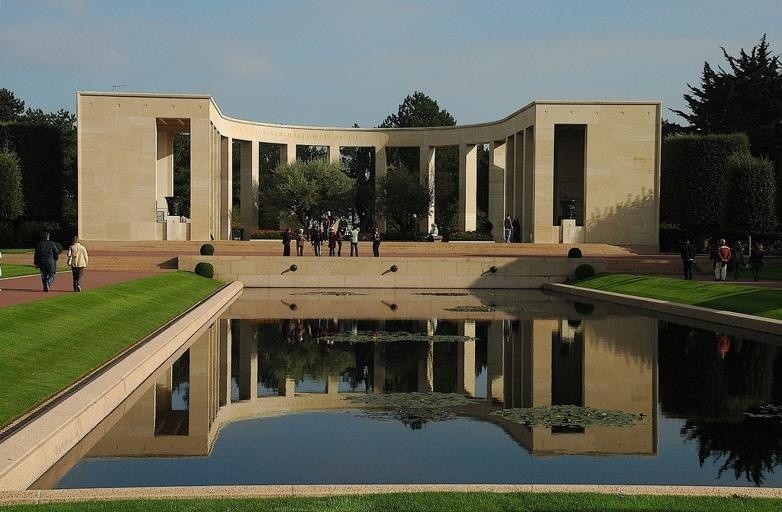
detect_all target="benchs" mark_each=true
[428,235,443,242]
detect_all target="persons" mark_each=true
[680,238,697,280]
[282,316,359,346]
[503,213,521,243]
[281,222,360,257]
[428,223,438,242]
[708,238,765,283]
[718,335,730,361]
[370,227,382,257]
[410,213,420,242]
[66,236,89,292]
[425,223,435,240]
[34,231,60,291]
